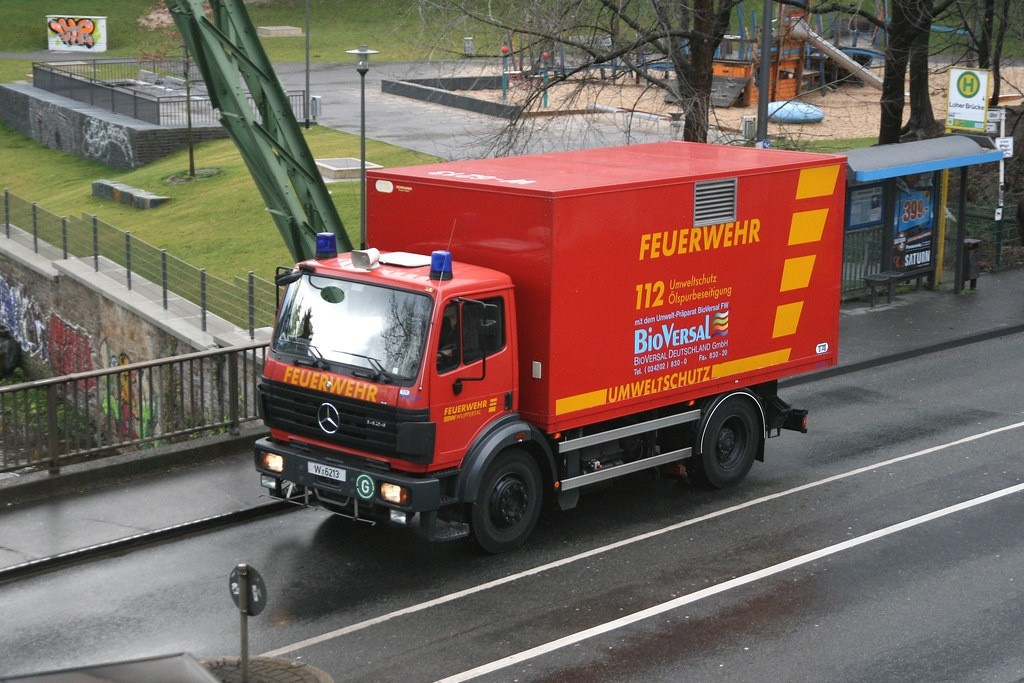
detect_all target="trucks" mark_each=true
[254,140,847,556]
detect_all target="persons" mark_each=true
[1016,198,1024,246]
[438,301,479,356]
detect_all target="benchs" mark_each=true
[860,269,905,308]
[150,76,187,96]
[125,69,159,90]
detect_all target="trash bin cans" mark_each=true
[962,239,980,290]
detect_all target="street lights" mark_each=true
[345,44,380,250]
